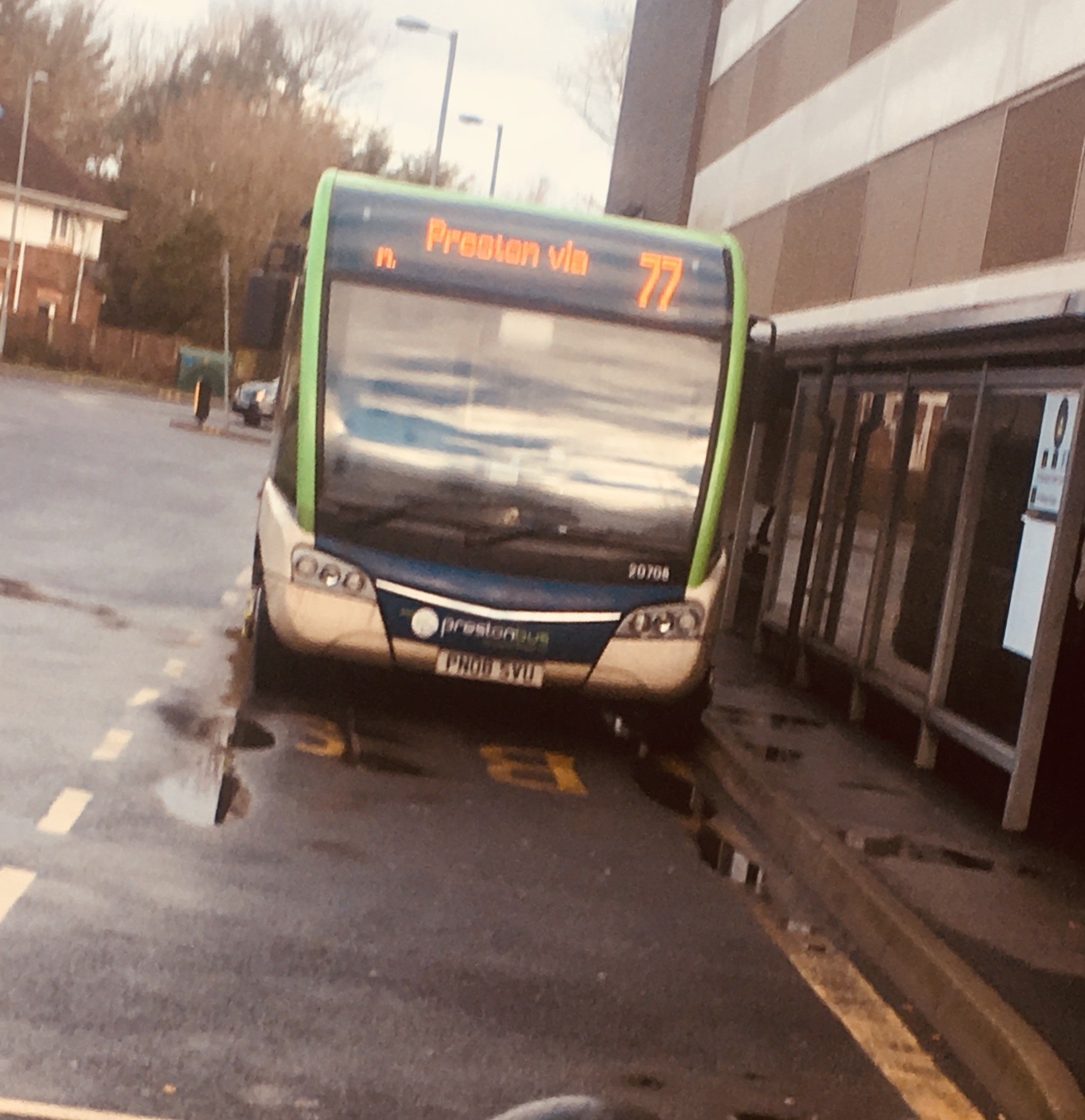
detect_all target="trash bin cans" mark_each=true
[174,346,234,398]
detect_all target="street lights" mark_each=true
[0,69,51,359]
[459,112,503,199]
[394,13,458,188]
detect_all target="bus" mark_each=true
[251,167,777,760]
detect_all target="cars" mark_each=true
[231,376,280,428]
